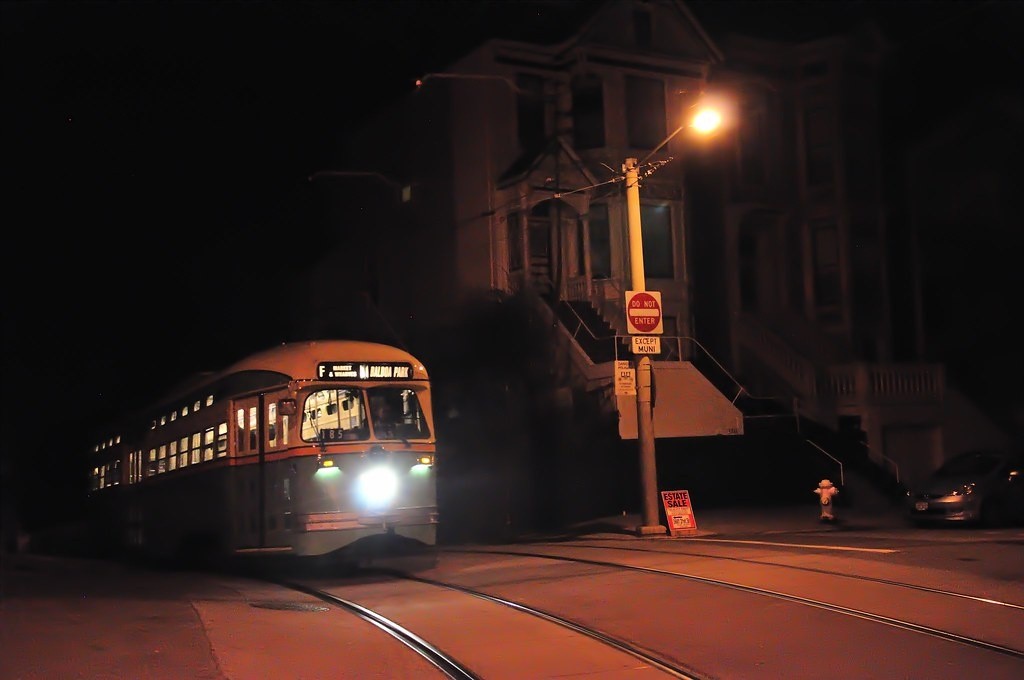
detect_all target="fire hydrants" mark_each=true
[815,479,839,522]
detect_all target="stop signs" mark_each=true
[625,291,663,334]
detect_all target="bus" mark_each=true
[83,340,444,584]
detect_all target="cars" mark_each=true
[914,458,1024,526]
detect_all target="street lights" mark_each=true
[615,92,733,533]
